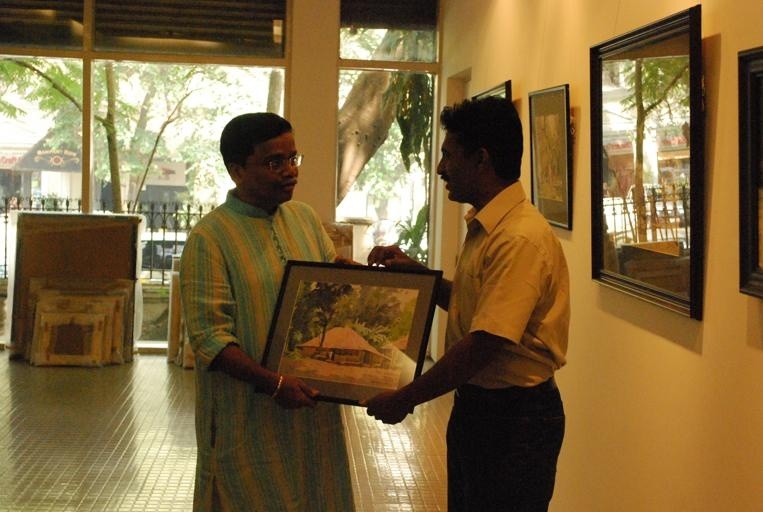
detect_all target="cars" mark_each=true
[142,232,190,280]
[603,183,691,248]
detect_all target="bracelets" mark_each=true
[270,375,283,397]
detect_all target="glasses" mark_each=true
[243,152,302,175]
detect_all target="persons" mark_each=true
[179,112,364,510]
[363,94,572,510]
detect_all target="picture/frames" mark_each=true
[527,82,576,233]
[585,2,707,323]
[8,212,141,369]
[471,76,514,101]
[166,254,196,369]
[253,260,444,414]
[734,41,763,304]
